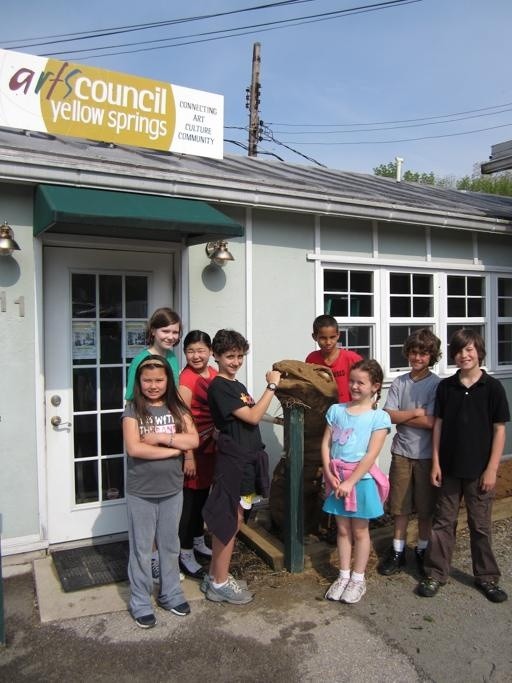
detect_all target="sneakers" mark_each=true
[418,576,441,596]
[340,578,366,603]
[135,548,255,628]
[480,581,507,601]
[327,576,349,599]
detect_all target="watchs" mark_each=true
[266,382,277,390]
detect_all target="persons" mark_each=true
[200,329,282,604]
[128,306,186,585]
[322,358,393,602]
[304,314,365,403]
[418,327,509,602]
[122,354,200,628]
[382,328,443,577]
[177,330,218,579]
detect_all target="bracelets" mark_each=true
[165,431,176,446]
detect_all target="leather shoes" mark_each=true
[382,545,405,574]
[415,546,425,575]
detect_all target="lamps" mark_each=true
[0,220,22,256]
[204,238,234,266]
